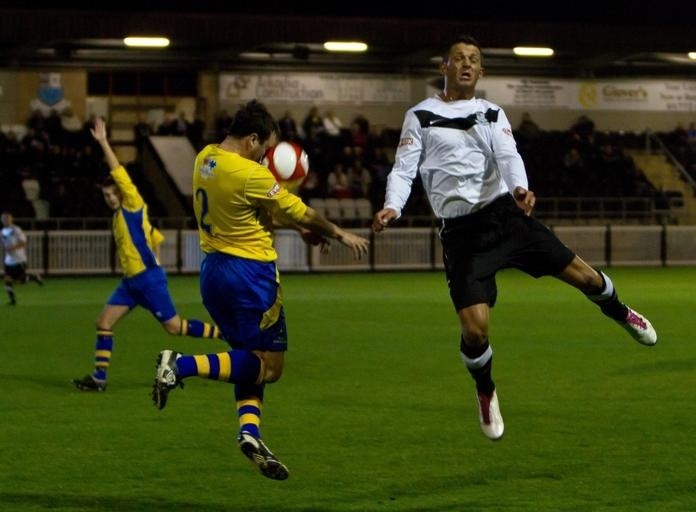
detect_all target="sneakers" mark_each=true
[239,435,289,481]
[6,299,17,305]
[35,273,43,287]
[72,376,106,393]
[610,305,657,346]
[152,350,182,409]
[474,383,504,441]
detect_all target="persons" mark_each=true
[151,99,371,479]
[278,107,431,225]
[70,117,227,390]
[371,36,658,439]
[0,213,44,305]
[0,110,232,218]
[514,111,650,217]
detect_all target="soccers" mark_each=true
[261,142,309,189]
